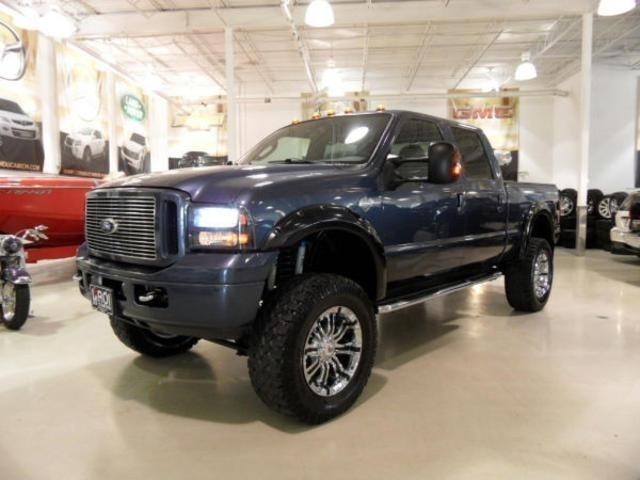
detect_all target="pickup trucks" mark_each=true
[74,109,560,424]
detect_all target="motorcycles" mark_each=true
[0,224,48,331]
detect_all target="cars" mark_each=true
[119,132,151,173]
[178,151,227,168]
[609,188,640,258]
[0,97,41,142]
[64,127,106,163]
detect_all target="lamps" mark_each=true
[514,50,538,82]
[303,0,335,29]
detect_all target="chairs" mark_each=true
[398,145,427,176]
